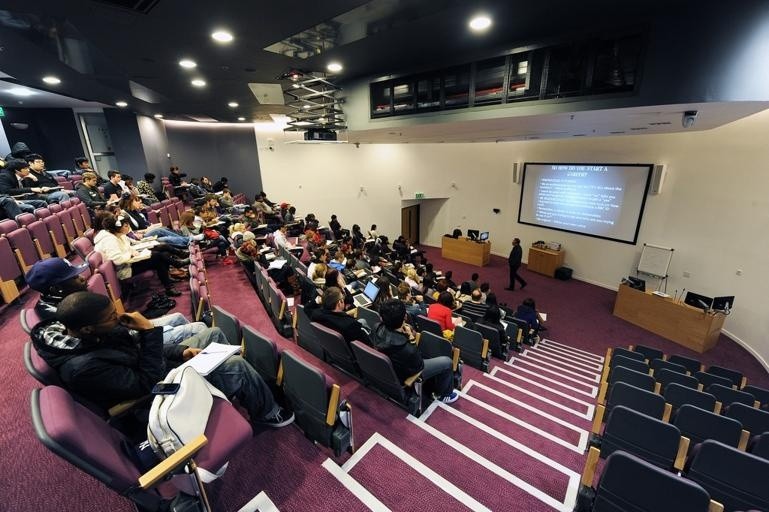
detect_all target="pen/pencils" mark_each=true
[201,350,228,354]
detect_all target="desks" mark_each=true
[527,245,566,278]
[441,234,491,268]
[612,281,726,354]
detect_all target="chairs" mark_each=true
[699,365,747,389]
[645,358,686,377]
[600,355,649,379]
[365,266,374,278]
[85,250,129,315]
[661,355,701,373]
[188,263,210,296]
[294,268,306,292]
[314,287,327,298]
[738,430,769,460]
[260,271,277,315]
[356,306,382,329]
[452,325,492,372]
[697,383,754,410]
[452,310,474,329]
[83,228,99,244]
[68,173,82,188]
[374,273,381,281]
[174,225,218,262]
[506,311,541,346]
[187,254,208,277]
[232,192,248,207]
[592,382,666,426]
[753,400,769,412]
[474,322,511,362]
[628,345,663,363]
[71,237,154,285]
[648,367,698,390]
[417,330,464,390]
[15,212,55,261]
[350,339,425,417]
[229,244,241,254]
[713,400,769,436]
[60,199,89,240]
[398,278,407,285]
[297,261,308,277]
[70,263,110,304]
[238,214,262,246]
[70,196,94,233]
[188,277,211,325]
[502,319,524,353]
[47,203,80,251]
[675,436,768,511]
[402,309,413,325]
[18,309,48,337]
[328,225,338,242]
[588,403,679,475]
[0,237,23,315]
[0,218,39,273]
[386,271,397,283]
[253,261,267,293]
[574,446,710,512]
[303,277,316,299]
[151,202,170,228]
[188,245,204,263]
[462,309,486,324]
[686,370,734,390]
[277,243,284,258]
[53,176,73,190]
[281,348,354,455]
[605,348,645,364]
[283,248,291,265]
[267,232,297,258]
[415,315,443,337]
[160,174,176,198]
[239,323,284,395]
[209,305,244,356]
[170,195,186,219]
[267,282,298,337]
[234,251,281,286]
[24,340,166,428]
[30,384,253,512]
[295,302,326,361]
[310,321,363,380]
[411,285,423,298]
[33,207,66,258]
[142,206,157,225]
[162,198,180,228]
[661,402,741,450]
[290,255,299,271]
[653,381,716,414]
[424,294,438,305]
[388,282,399,297]
[597,366,655,405]
[733,384,769,403]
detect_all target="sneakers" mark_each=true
[437,392,458,404]
[256,403,296,427]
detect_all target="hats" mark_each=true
[280,201,289,210]
[24,257,88,291]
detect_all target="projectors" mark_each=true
[303,130,338,143]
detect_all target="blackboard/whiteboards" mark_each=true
[637,242,674,279]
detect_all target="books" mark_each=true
[129,249,151,265]
[179,342,243,378]
[269,260,287,269]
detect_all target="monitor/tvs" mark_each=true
[629,276,646,292]
[684,291,713,314]
[480,232,489,242]
[712,296,735,315]
[467,229,479,241]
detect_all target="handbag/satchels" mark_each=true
[147,365,229,483]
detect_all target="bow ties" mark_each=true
[115,215,124,227]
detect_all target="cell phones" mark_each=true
[152,383,180,395]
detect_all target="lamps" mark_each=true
[0,105,7,119]
[167,153,172,159]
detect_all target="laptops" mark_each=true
[353,280,380,308]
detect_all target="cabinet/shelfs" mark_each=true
[372,53,534,115]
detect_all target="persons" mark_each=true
[74,172,107,209]
[368,298,459,404]
[5,142,29,162]
[121,174,140,197]
[99,201,193,247]
[192,176,296,261]
[32,291,294,429]
[481,282,497,306]
[25,154,70,204]
[0,159,48,213]
[468,273,479,291]
[478,304,509,345]
[523,298,547,330]
[439,271,457,292]
[456,281,471,303]
[432,280,449,301]
[169,164,188,198]
[396,287,427,329]
[466,290,486,304]
[25,257,208,349]
[427,292,455,330]
[104,169,123,199]
[138,172,160,203]
[504,238,528,292]
[70,157,109,186]
[298,214,437,287]
[304,287,362,341]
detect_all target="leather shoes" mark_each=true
[174,258,190,269]
[166,285,181,296]
